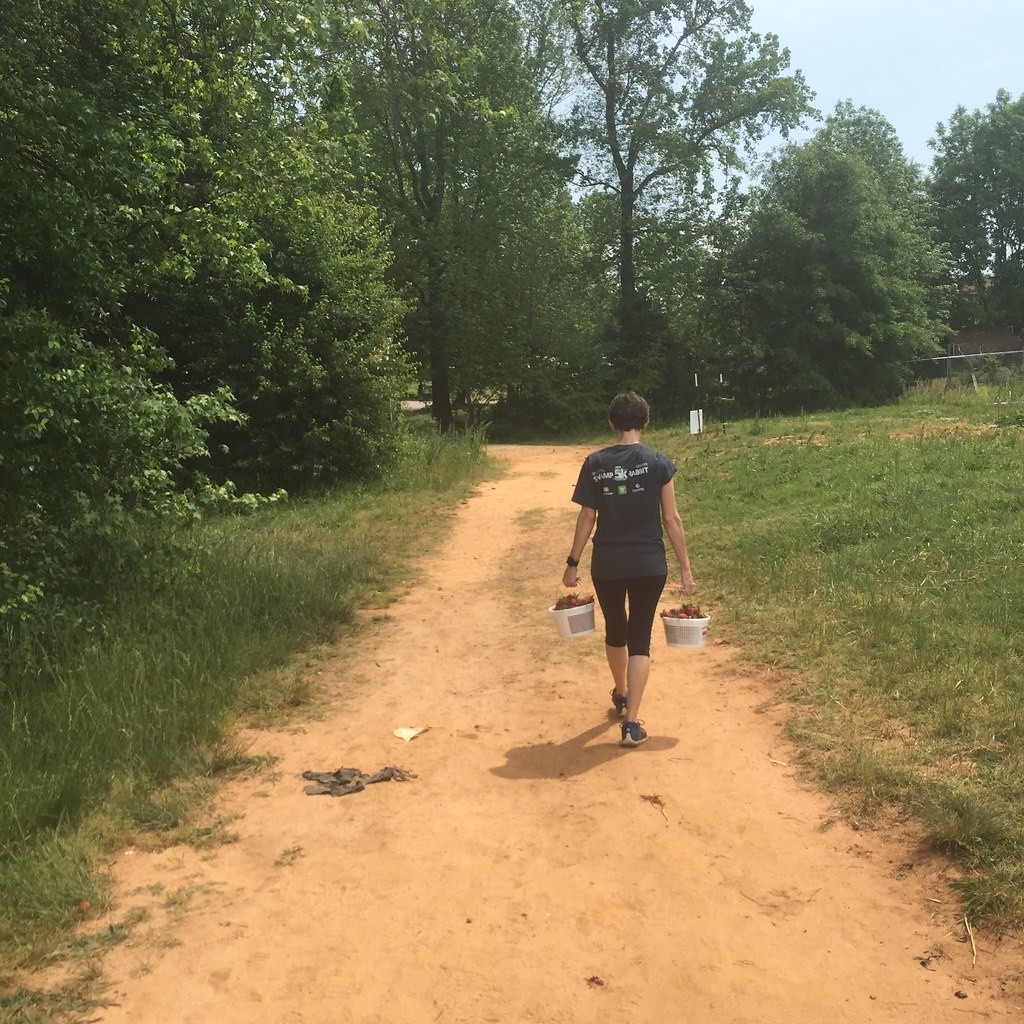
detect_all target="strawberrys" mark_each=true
[557,593,594,609]
[660,604,706,619]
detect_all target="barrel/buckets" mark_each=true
[661,590,710,648]
[548,578,596,640]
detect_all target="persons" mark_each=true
[562,391,696,747]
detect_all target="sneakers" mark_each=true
[619,719,648,745]
[609,688,627,713]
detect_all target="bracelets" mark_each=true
[565,556,580,567]
